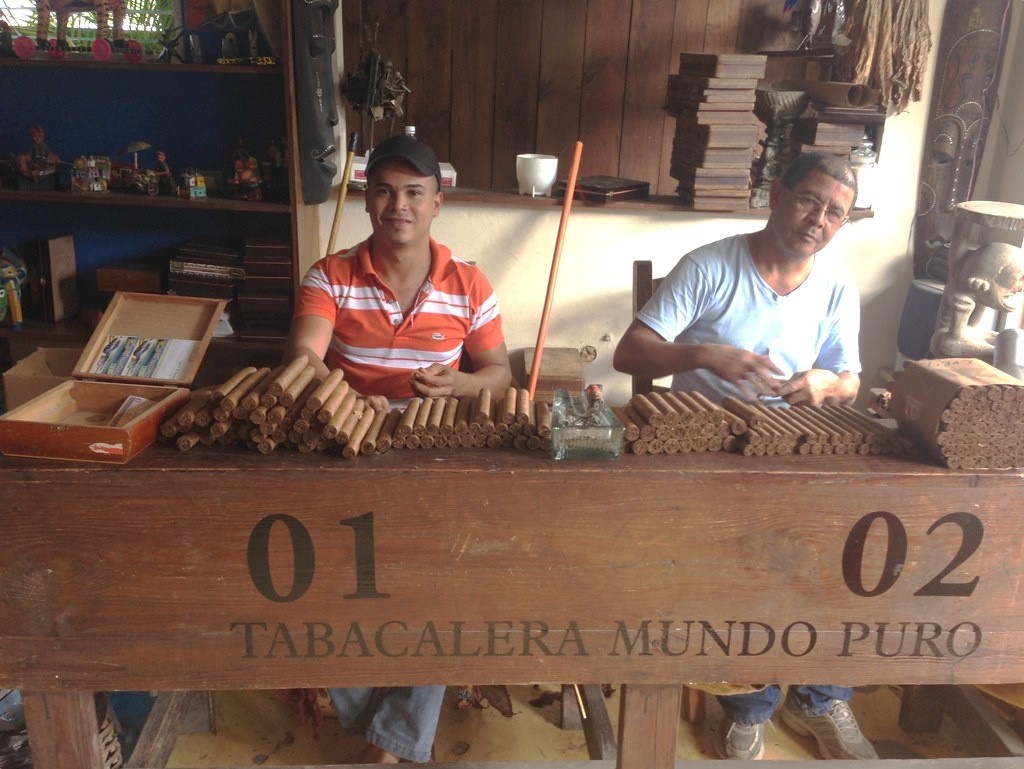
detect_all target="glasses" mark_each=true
[791,191,847,227]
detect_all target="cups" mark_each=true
[515,153,559,198]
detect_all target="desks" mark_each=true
[0,415,1023,768]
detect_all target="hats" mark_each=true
[364,135,441,192]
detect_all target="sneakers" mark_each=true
[780,691,879,760]
[714,714,766,761]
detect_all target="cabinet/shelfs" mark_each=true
[0,0,302,345]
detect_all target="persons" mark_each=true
[613,150,881,760]
[233,157,262,200]
[17,125,60,182]
[283,136,511,763]
[154,151,177,195]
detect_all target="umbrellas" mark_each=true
[118,140,151,169]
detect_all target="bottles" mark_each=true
[405,125,417,141]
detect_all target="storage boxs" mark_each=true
[0,235,290,463]
[667,53,864,212]
[524,346,586,397]
[558,176,650,206]
